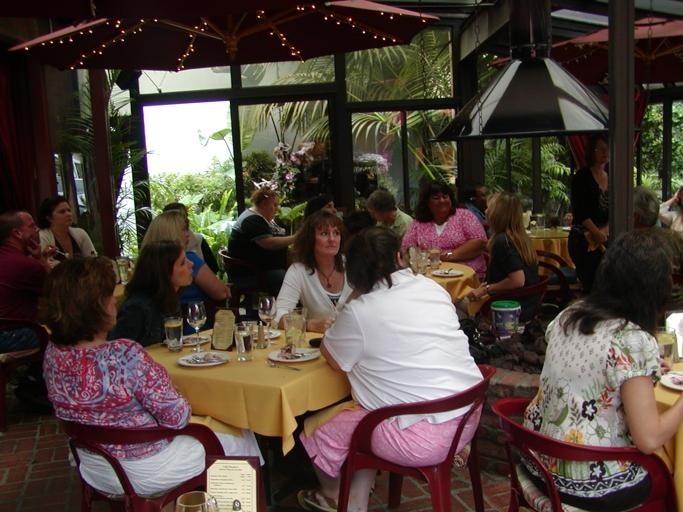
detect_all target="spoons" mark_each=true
[264,358,299,371]
[443,268,453,273]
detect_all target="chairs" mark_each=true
[1,318,52,432]
[337,364,496,512]
[491,394,681,511]
[219,250,276,322]
[56,419,228,512]
[473,249,585,356]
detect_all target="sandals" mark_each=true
[297,489,339,512]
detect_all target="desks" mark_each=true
[646,334,681,492]
[109,276,129,310]
[522,226,577,274]
[408,259,482,326]
[140,323,352,494]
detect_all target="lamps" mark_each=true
[424,0,646,149]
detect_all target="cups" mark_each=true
[409,235,440,274]
[490,300,521,336]
[283,315,305,345]
[654,330,677,366]
[233,322,254,361]
[287,307,307,346]
[529,221,538,234]
[163,316,183,351]
[114,255,131,284]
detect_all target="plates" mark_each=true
[660,372,682,391]
[252,329,280,341]
[163,336,209,346]
[431,270,463,277]
[177,352,230,366]
[561,227,571,231]
[268,348,321,363]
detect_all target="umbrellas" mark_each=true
[8,1,440,219]
[489,17,682,188]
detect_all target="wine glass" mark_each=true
[258,296,277,352]
[186,300,208,352]
